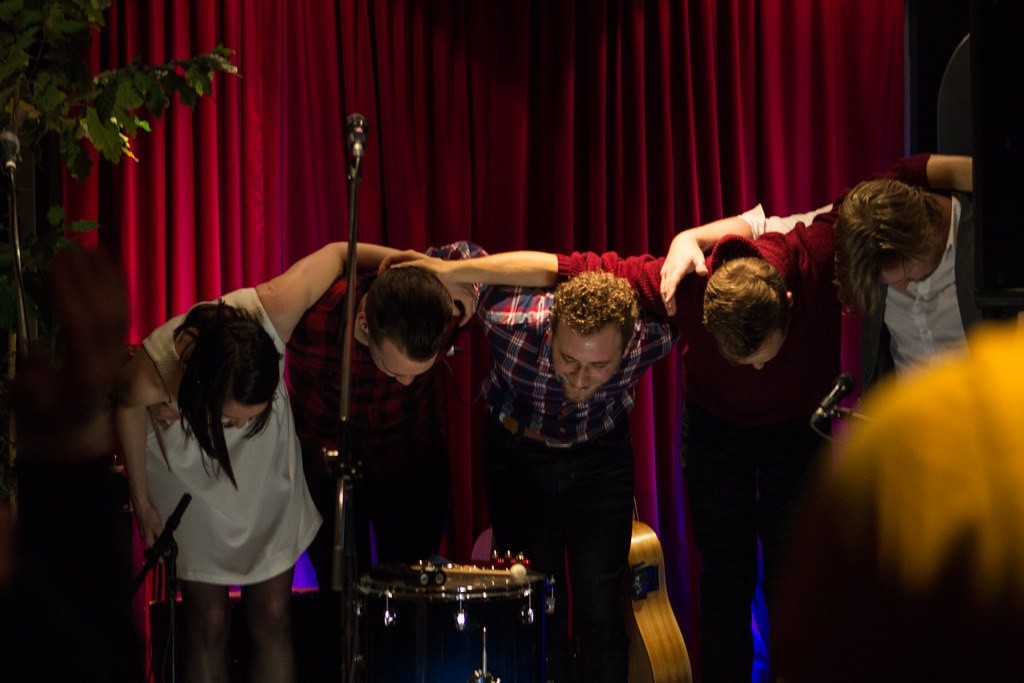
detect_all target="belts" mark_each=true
[489,406,574,446]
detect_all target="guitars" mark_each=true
[626,496,695,683]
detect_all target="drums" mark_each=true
[353,562,548,683]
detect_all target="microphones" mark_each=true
[342,113,369,181]
[0,132,21,191]
[810,374,857,425]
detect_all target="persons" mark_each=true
[376,153,973,683]
[285,240,468,683]
[659,178,1024,398]
[112,240,477,683]
[770,311,1024,683]
